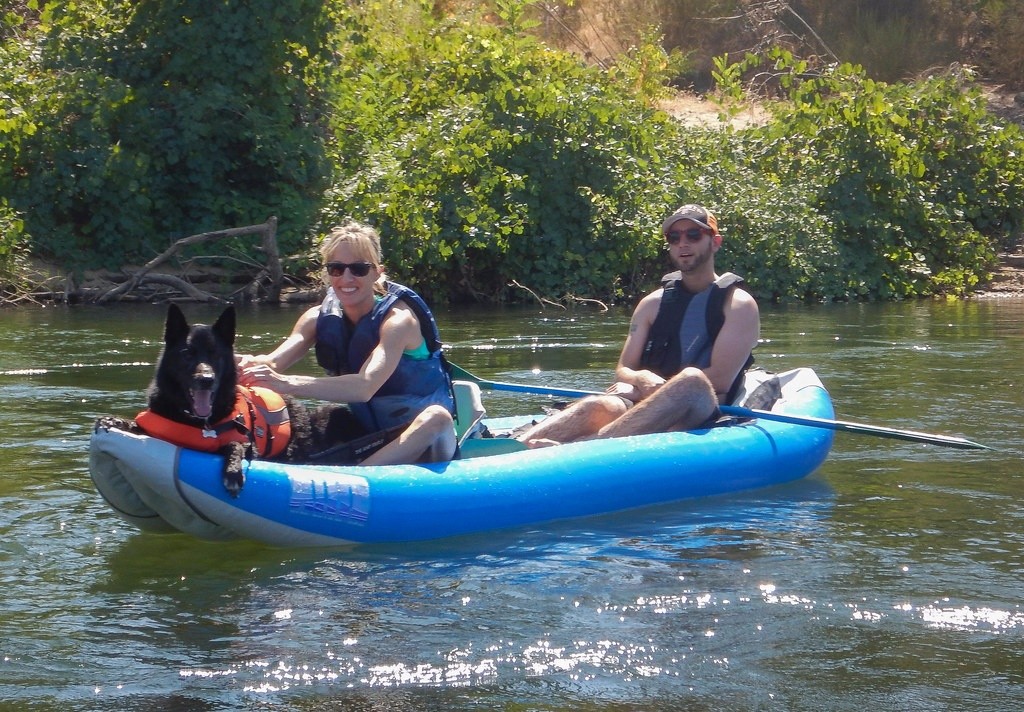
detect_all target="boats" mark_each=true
[83,361,838,555]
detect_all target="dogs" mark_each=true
[94,301,365,501]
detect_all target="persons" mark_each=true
[516,204,762,448]
[233,225,460,466]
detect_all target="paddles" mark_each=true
[446,359,992,452]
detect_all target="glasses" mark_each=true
[665,228,714,244]
[324,263,374,276]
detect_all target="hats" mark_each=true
[662,204,717,236]
